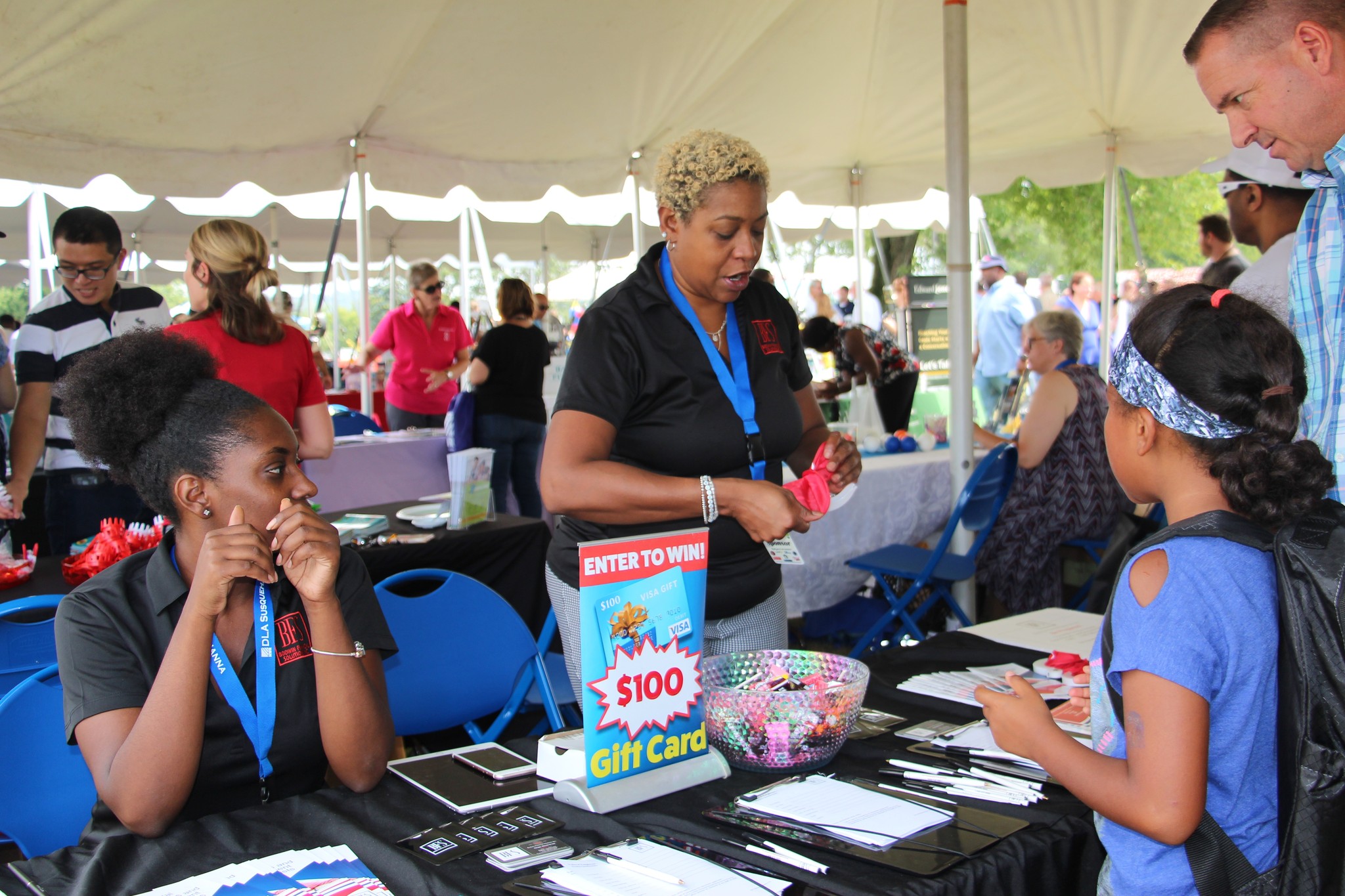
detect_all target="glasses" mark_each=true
[1026,336,1045,348]
[417,281,445,294]
[536,305,549,311]
[1216,180,1265,199]
[53,255,116,281]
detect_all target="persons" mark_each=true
[57,334,403,854]
[801,317,919,434]
[535,291,566,357]
[340,261,477,431]
[466,276,551,522]
[971,286,1310,896]
[1182,0,1345,510]
[1216,141,1317,331]
[752,266,800,327]
[974,255,1159,430]
[974,309,1138,624]
[809,279,854,320]
[539,134,867,728]
[1,206,339,561]
[1195,215,1249,289]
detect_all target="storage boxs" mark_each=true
[537,728,586,783]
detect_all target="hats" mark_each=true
[978,256,1010,274]
[1199,138,1314,190]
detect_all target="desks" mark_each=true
[0,498,552,643]
[0,607,1107,896]
[779,445,989,618]
[323,388,390,432]
[297,427,558,533]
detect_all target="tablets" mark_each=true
[385,747,557,814]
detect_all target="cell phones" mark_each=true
[451,746,537,781]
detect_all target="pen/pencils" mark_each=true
[720,837,821,875]
[741,832,833,875]
[877,745,1050,806]
[585,849,683,884]
[766,679,789,692]
[789,677,810,691]
[734,672,762,690]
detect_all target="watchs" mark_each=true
[444,369,453,381]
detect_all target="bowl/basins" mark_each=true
[702,650,870,774]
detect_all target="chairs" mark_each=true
[0,595,66,842]
[373,568,583,744]
[844,442,1018,660]
[331,411,382,437]
[1063,501,1165,611]
[0,663,97,860]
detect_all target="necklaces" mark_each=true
[657,257,727,351]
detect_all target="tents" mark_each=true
[0,174,1000,395]
[0,0,1345,426]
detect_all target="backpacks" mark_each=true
[1098,499,1345,896]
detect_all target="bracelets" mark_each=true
[700,474,719,524]
[311,641,366,658]
